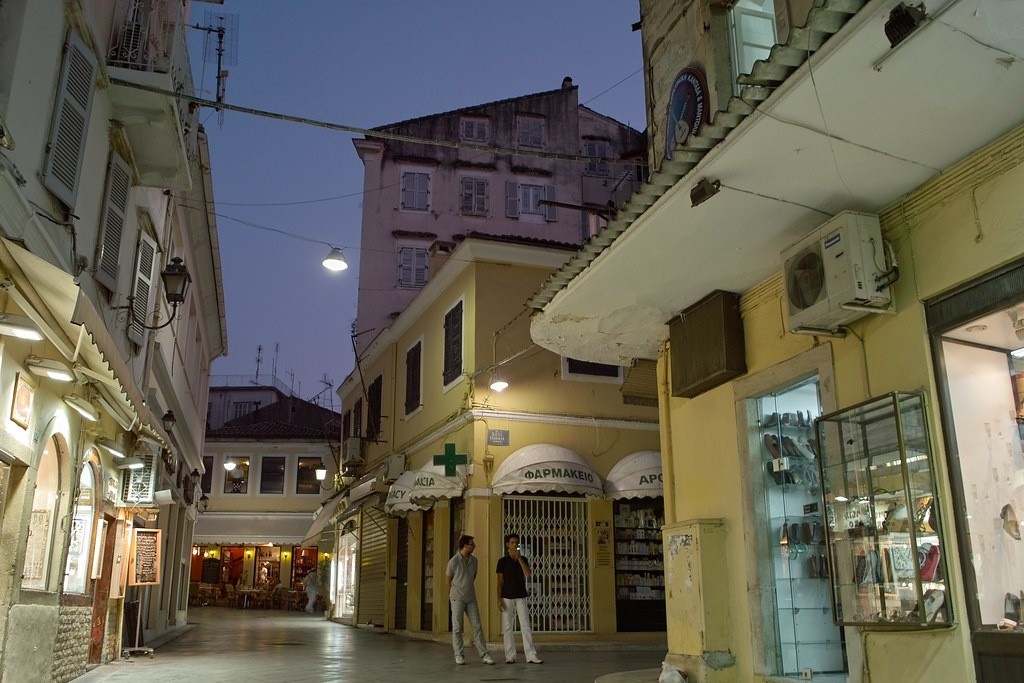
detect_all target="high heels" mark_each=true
[1000,502,1022,542]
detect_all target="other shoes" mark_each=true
[505,658,515,664]
[763,411,828,579]
[528,656,544,664]
[992,590,1024,632]
[851,498,946,626]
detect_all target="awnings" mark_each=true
[385,458,468,516]
[490,443,604,498]
[300,490,348,549]
[0,234,177,460]
[604,451,663,501]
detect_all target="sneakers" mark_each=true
[455,655,465,664]
[483,654,495,664]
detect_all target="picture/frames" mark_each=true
[11,372,38,430]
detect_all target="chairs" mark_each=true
[185,579,321,612]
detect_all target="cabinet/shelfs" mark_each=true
[814,389,954,632]
[761,422,845,647]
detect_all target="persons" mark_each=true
[446,535,496,664]
[230,465,244,493]
[303,569,319,615]
[259,563,268,582]
[496,534,544,664]
[223,565,229,581]
[260,577,284,608]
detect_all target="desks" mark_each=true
[289,590,309,609]
[236,589,261,608]
[197,587,219,605]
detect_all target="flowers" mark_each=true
[147,31,167,58]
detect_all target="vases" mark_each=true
[154,55,170,74]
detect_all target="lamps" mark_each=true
[25,356,74,381]
[689,178,722,208]
[490,371,508,391]
[181,468,202,491]
[322,246,351,270]
[195,495,211,513]
[61,395,100,422]
[224,457,236,470]
[0,311,48,341]
[315,463,338,490]
[193,544,215,559]
[114,457,144,473]
[110,257,192,331]
[94,438,127,456]
[162,409,176,435]
[247,550,252,558]
[284,552,289,558]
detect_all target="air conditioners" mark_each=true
[382,455,407,482]
[341,437,364,465]
[116,20,149,72]
[776,207,897,338]
[127,452,166,504]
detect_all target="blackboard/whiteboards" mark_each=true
[128,528,162,586]
